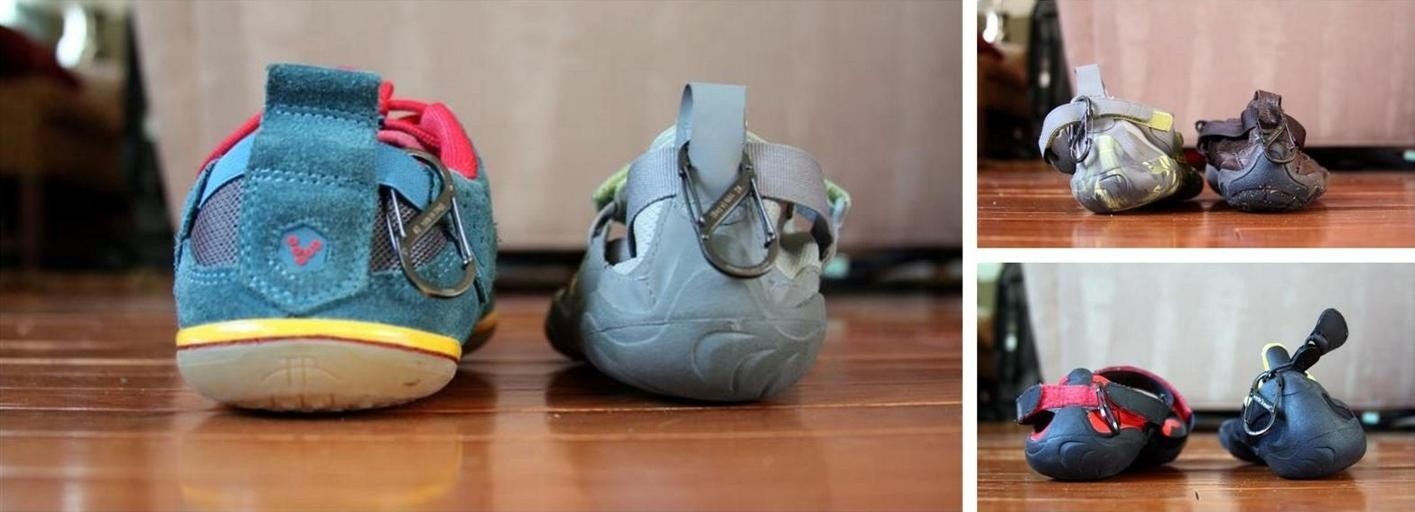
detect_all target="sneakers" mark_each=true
[1194,83,1330,213]
[1015,362,1197,481]
[542,76,854,403]
[170,63,501,417]
[1036,62,1204,215]
[1218,309,1368,480]
